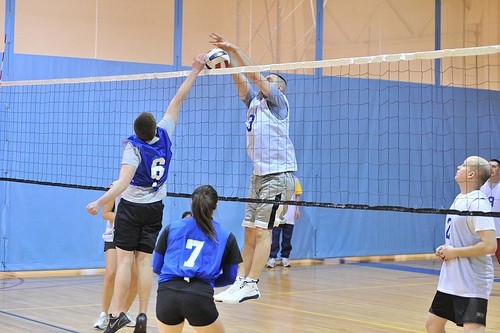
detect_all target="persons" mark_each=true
[182,211,193,219]
[209,32,298,304]
[92,180,139,330]
[423,156,497,333]
[84,52,210,333]
[479,159,500,264]
[266,174,304,268]
[150,185,244,333]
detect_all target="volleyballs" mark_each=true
[203,47,231,69]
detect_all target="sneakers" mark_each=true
[281,258,291,267]
[133,313,147,333]
[125,311,136,327]
[103,312,131,333]
[94,312,109,329]
[266,258,276,267]
[213,277,260,304]
